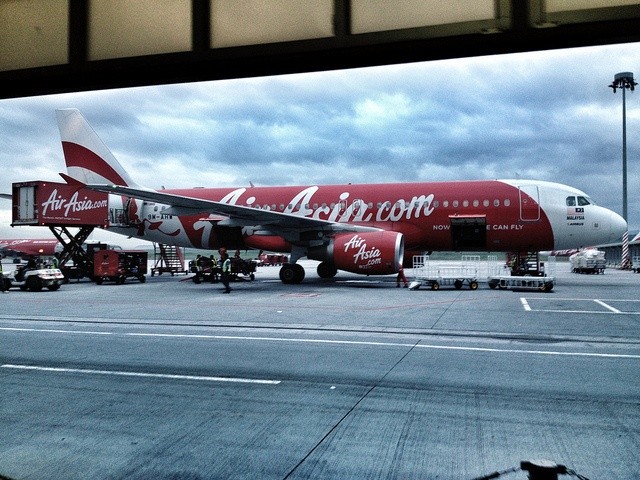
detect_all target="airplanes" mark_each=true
[10,108,628,291]
[0,239,120,259]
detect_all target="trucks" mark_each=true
[409,255,481,292]
[487,254,556,290]
[569,249,606,275]
[95,249,148,286]
[189,256,257,284]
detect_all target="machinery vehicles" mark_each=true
[150,244,188,276]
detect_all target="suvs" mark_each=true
[0,265,65,291]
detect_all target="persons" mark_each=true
[19,254,38,272]
[396,263,409,289]
[51,251,60,268]
[221,253,233,294]
